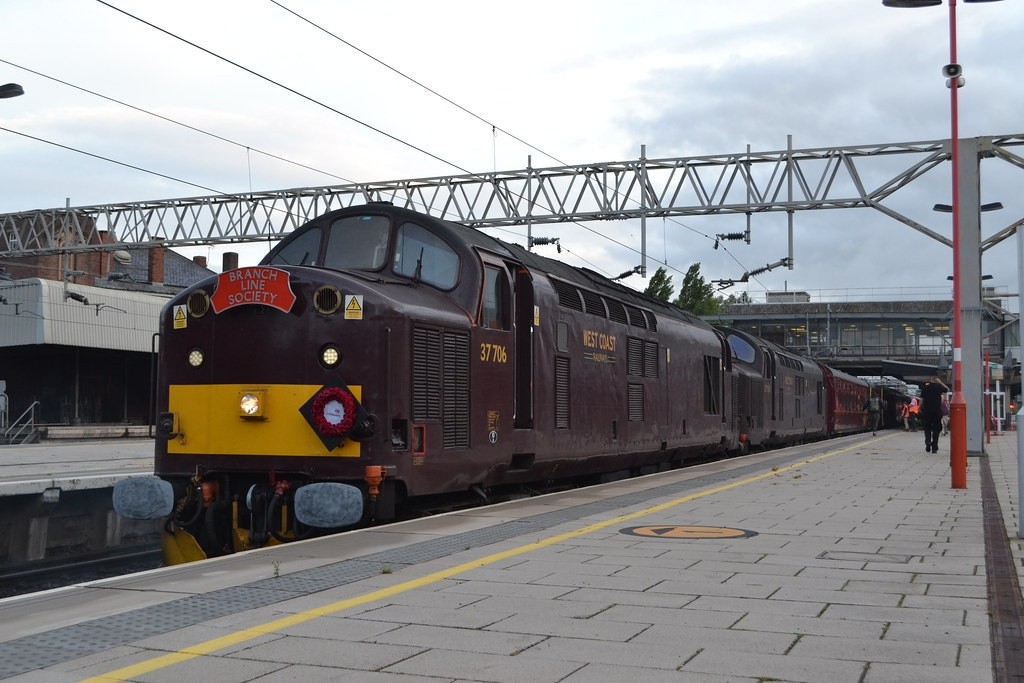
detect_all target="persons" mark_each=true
[908,397,920,432]
[920,378,949,453]
[901,401,910,431]
[863,391,883,436]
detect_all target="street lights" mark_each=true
[880,0,1005,488]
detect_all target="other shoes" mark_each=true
[926,442,930,452]
[932,450,937,453]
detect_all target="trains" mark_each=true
[110,203,921,567]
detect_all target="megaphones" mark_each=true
[945,77,965,89]
[942,64,962,77]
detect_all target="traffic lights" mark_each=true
[1010,404,1015,409]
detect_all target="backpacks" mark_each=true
[941,401,948,416]
